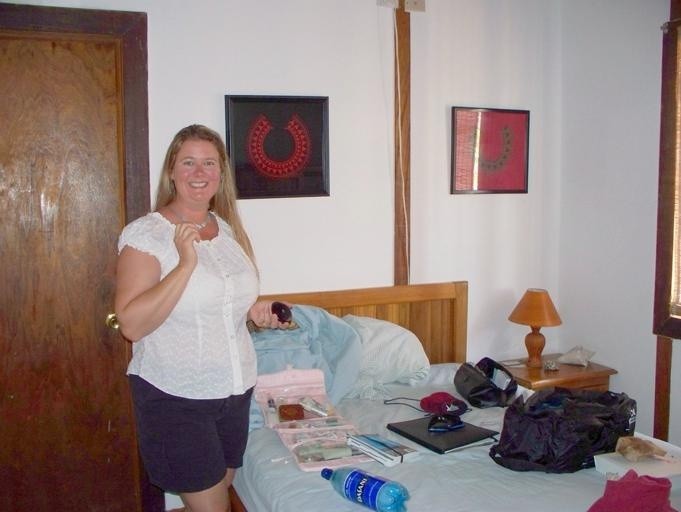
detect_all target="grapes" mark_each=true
[495,352,618,395]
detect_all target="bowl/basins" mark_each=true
[169,204,211,230]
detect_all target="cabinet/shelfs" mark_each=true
[320,466,408,512]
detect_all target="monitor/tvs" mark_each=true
[593,452,681,480]
[348,432,421,468]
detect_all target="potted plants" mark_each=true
[223,93,332,201]
[451,104,531,196]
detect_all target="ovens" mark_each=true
[228,279,681,512]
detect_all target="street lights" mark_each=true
[453,358,518,407]
[490,385,637,474]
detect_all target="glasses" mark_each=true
[508,288,563,367]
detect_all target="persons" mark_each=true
[114,124,299,512]
